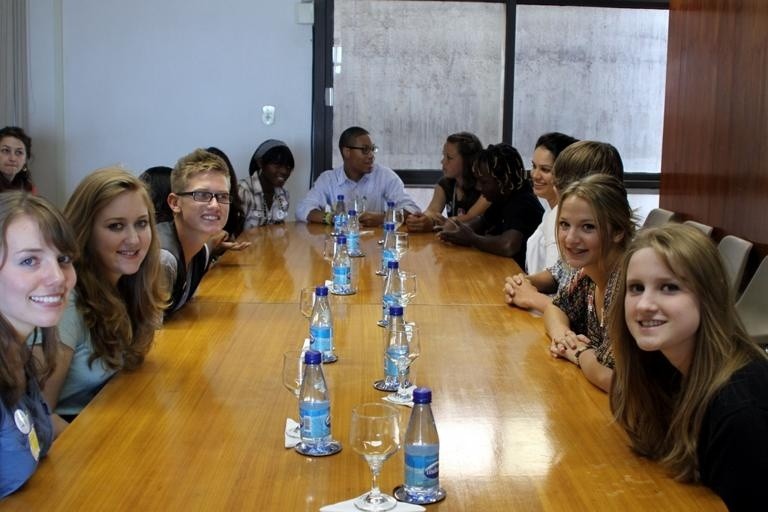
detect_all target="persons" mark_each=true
[237,139,294,230]
[522,132,580,276]
[542,172,641,393]
[405,132,494,236]
[293,126,421,227]
[430,143,543,274]
[604,221,767,511]
[206,147,253,256]
[24,166,174,442]
[154,148,232,328]
[502,138,627,314]
[0,126,37,196]
[0,187,81,501]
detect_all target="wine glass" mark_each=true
[323,231,340,285]
[300,289,327,329]
[282,349,307,438]
[383,229,409,272]
[340,195,409,268]
[391,270,417,319]
[385,321,421,403]
[349,402,401,512]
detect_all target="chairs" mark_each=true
[634,207,768,366]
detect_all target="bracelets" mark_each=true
[321,212,331,225]
[573,344,597,369]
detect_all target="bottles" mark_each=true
[298,350,331,454]
[385,306,409,388]
[330,234,351,296]
[404,387,440,500]
[382,260,402,325]
[308,287,333,361]
[329,194,398,274]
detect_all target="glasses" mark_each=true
[176,192,233,204]
[345,145,380,155]
